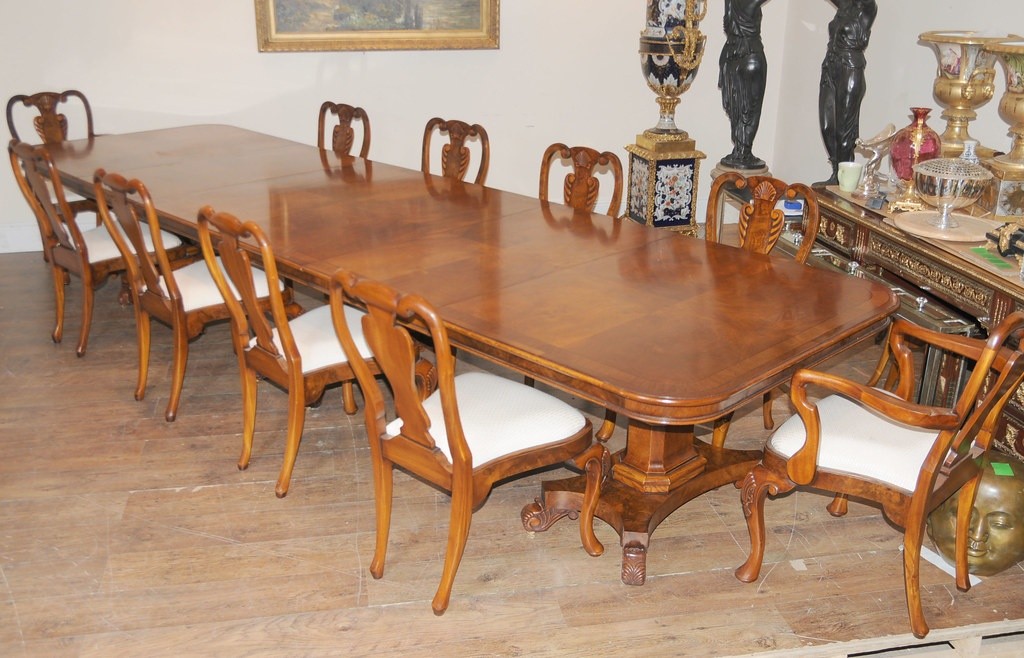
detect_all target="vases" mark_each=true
[919,29,1024,158]
[964,39,1024,222]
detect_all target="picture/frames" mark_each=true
[254,0,499,52]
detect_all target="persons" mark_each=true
[818,0,879,183]
[925,447,1024,577]
[718,0,770,164]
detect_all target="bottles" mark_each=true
[890,107,941,182]
[959,140,979,165]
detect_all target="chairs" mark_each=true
[420,116,489,185]
[8,140,187,359]
[317,100,371,157]
[7,90,113,285]
[595,171,819,447]
[197,205,437,498]
[330,269,610,616]
[92,169,306,422]
[451,142,624,389]
[734,309,1024,639]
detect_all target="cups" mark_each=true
[838,162,863,192]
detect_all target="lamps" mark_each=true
[618,0,708,236]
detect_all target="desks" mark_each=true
[791,178,1024,463]
[19,124,900,587]
